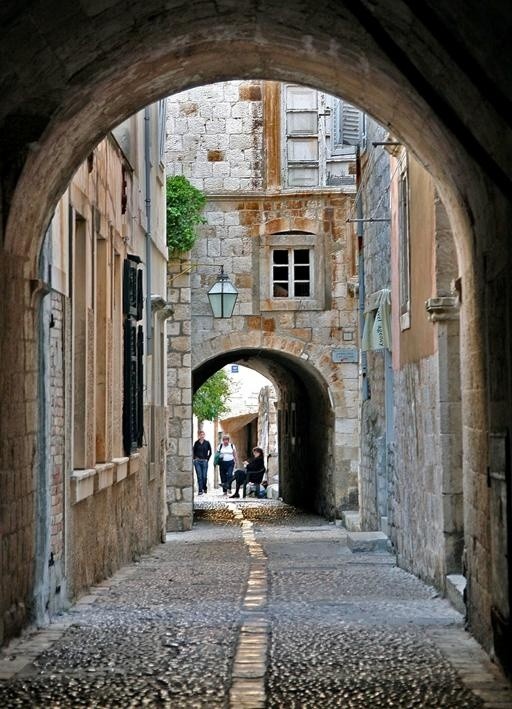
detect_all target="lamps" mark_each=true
[166,264,239,319]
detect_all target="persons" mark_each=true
[218,447,264,498]
[216,436,238,496]
[193,431,212,495]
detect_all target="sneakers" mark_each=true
[198,483,240,498]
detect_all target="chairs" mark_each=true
[243,468,264,498]
